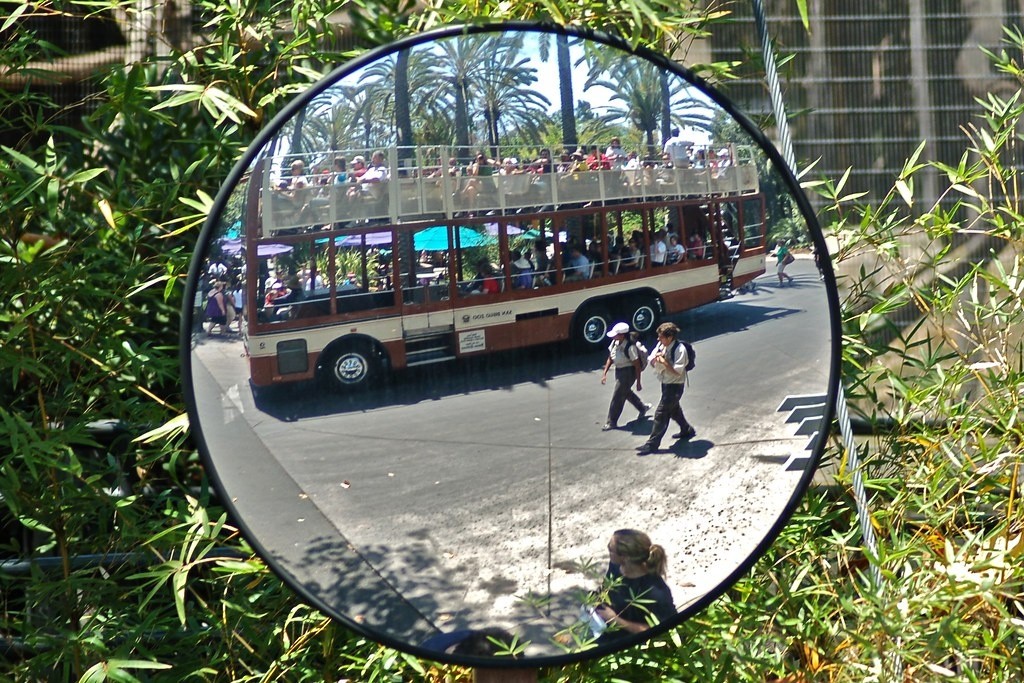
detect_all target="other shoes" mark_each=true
[636,443,658,452]
[601,423,617,430]
[776,284,783,287]
[672,426,695,438]
[787,278,793,285]
[638,403,652,418]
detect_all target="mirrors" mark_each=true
[177,19,845,669]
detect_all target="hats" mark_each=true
[606,322,630,337]
[350,155,365,164]
[716,149,727,157]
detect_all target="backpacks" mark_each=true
[624,341,648,371]
[658,341,695,370]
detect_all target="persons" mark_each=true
[601,322,649,431]
[811,243,822,280]
[771,239,793,288]
[595,529,679,644]
[635,322,695,452]
[205,127,733,338]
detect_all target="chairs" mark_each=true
[615,255,620,276]
[663,250,667,267]
[531,275,535,289]
[640,253,646,271]
[589,260,594,280]
[500,279,504,293]
[562,272,566,284]
[262,164,735,239]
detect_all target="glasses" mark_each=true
[608,543,616,554]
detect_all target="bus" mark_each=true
[241,142,766,393]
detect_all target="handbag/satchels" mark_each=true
[783,253,794,265]
